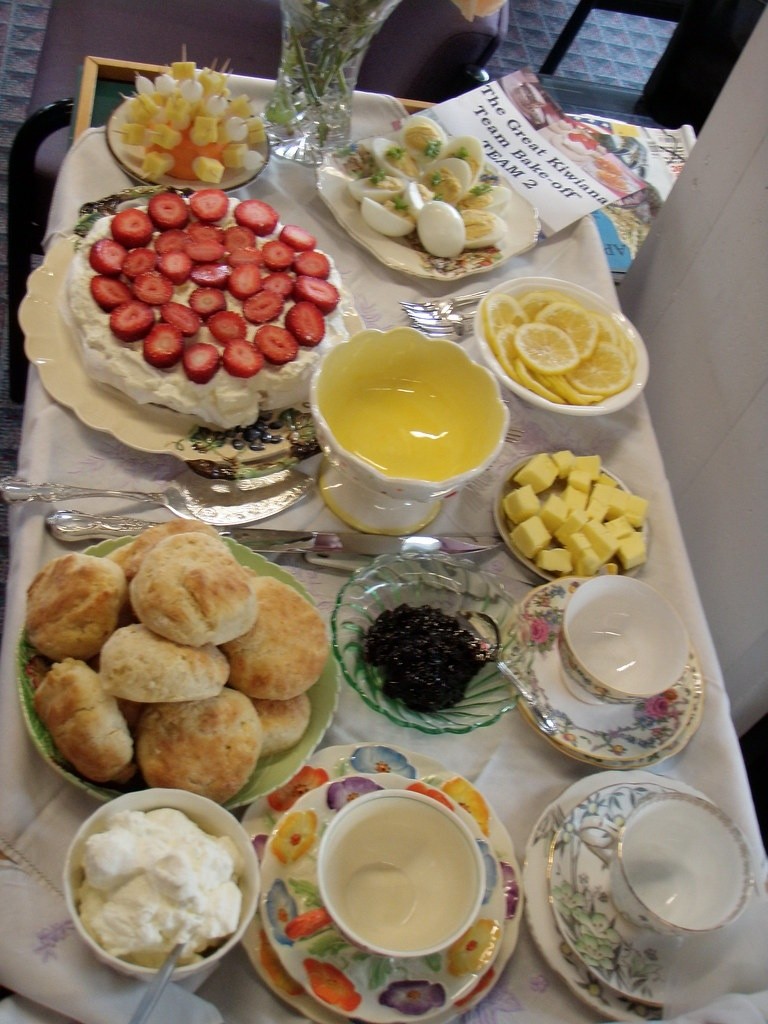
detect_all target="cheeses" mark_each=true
[504,448,648,578]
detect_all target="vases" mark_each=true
[253,0,402,165]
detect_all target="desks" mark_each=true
[2,56,768,1024]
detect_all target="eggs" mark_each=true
[346,116,510,257]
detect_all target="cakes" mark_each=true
[58,188,353,429]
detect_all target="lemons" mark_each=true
[481,289,638,406]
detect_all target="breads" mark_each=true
[23,518,330,805]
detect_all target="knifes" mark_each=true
[39,510,505,558]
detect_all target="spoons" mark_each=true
[0,470,317,525]
[455,610,561,735]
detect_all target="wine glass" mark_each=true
[309,326,512,537]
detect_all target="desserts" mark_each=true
[79,806,242,970]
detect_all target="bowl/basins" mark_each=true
[328,548,521,735]
[563,574,691,699]
[315,789,487,958]
[609,791,755,935]
[62,788,262,985]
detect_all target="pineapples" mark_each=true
[122,62,266,183]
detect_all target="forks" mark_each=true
[398,289,517,341]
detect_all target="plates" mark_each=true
[474,277,651,416]
[105,91,270,192]
[492,452,649,580]
[493,575,706,770]
[18,197,367,455]
[241,742,524,1024]
[14,535,342,811]
[522,770,768,1024]
[316,131,542,282]
